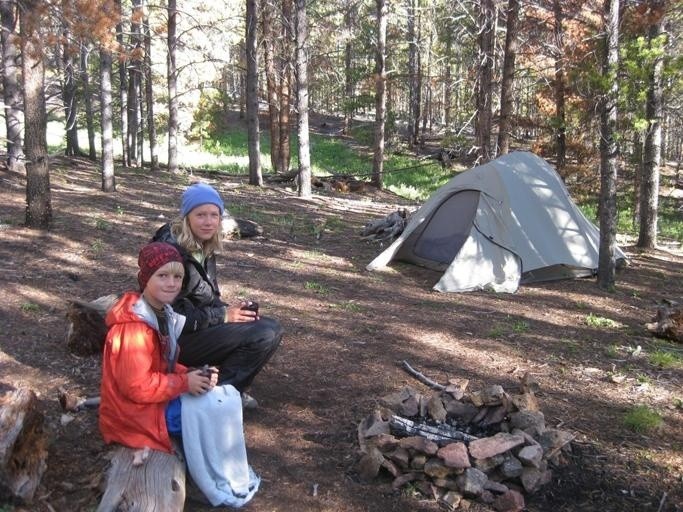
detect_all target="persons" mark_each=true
[151,183,284,411]
[97,244,243,453]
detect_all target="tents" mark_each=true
[364,150,630,295]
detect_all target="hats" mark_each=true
[182,175,224,217]
[137,241,185,294]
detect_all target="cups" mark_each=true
[199,369,212,386]
[243,301,259,319]
